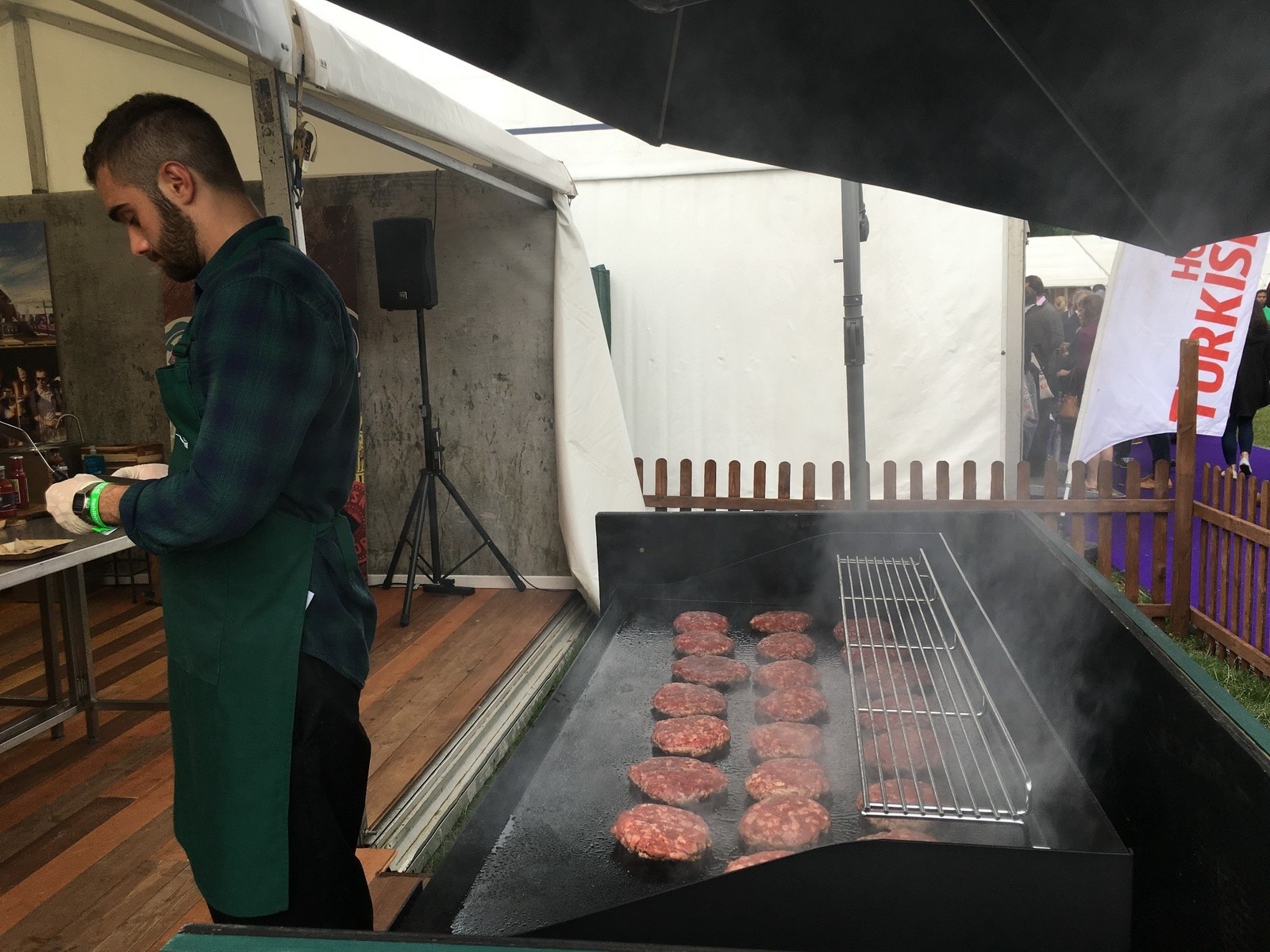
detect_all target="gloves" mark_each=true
[110,464,170,479]
[45,473,102,533]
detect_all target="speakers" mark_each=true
[373,216,439,312]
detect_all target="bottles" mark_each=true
[47,447,69,487]
[9,455,29,510]
[0,466,19,517]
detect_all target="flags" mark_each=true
[1073,232,1270,465]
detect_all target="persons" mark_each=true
[46,92,374,932]
[1022,275,1270,498]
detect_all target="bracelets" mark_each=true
[89,482,120,535]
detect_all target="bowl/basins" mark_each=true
[0,519,6,531]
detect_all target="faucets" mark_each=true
[54,414,85,443]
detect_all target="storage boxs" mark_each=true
[81,444,163,482]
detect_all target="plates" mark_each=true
[0,539,75,559]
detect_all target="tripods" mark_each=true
[380,310,527,627]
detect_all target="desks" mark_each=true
[0,515,137,754]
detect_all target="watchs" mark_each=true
[72,482,106,526]
